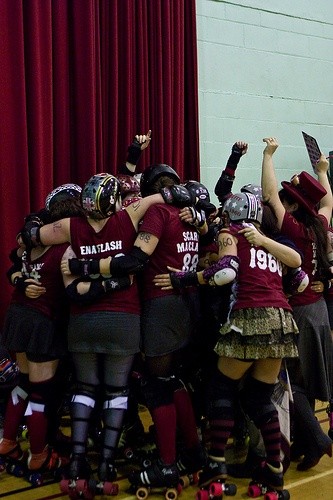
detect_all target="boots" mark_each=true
[296,416,332,470]
[288,419,320,459]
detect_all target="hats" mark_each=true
[281,171,327,216]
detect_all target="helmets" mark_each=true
[117,175,139,200]
[79,173,120,218]
[241,184,264,202]
[182,180,210,202]
[45,183,82,212]
[222,192,263,224]
[139,165,180,197]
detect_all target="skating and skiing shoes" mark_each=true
[0,424,291,500]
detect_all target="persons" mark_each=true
[0,130,333,488]
